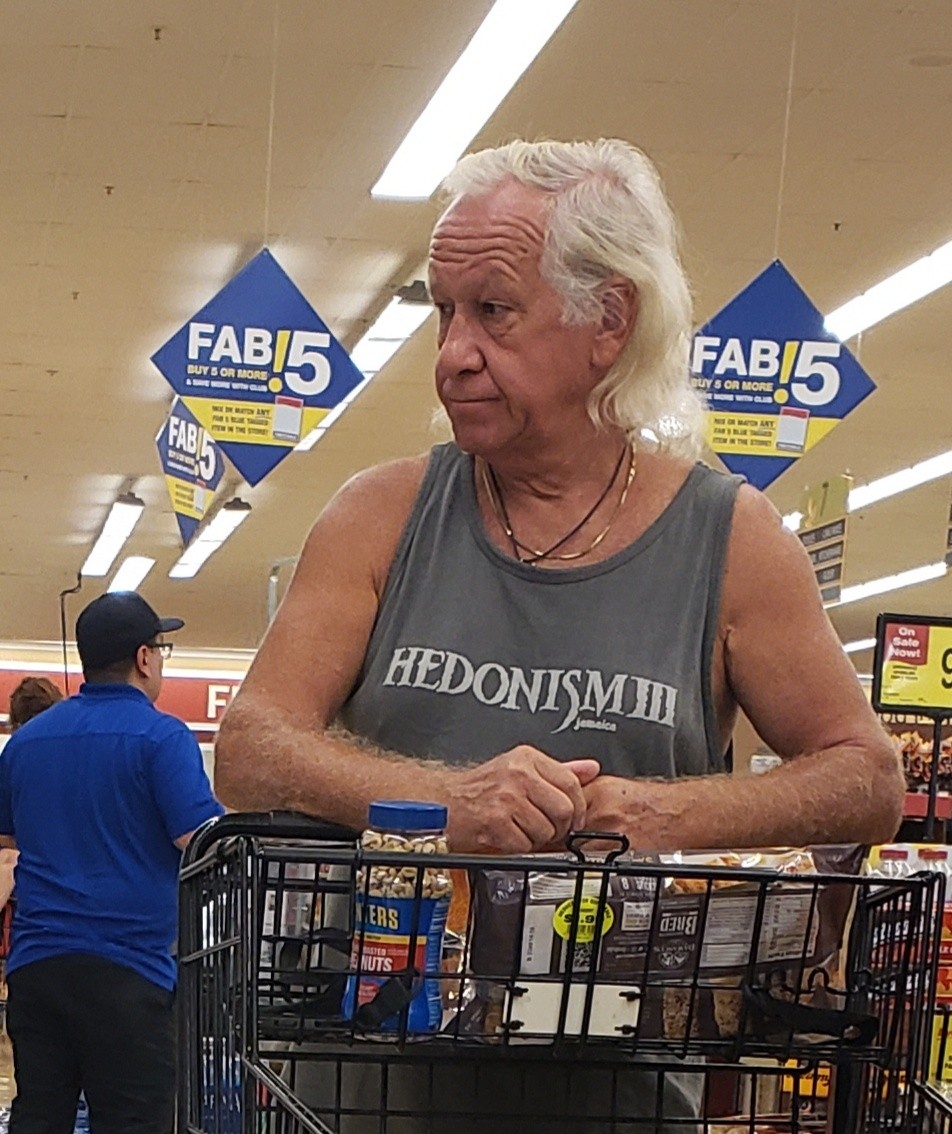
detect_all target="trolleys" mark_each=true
[175,812,952,1133]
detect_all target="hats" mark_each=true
[76,591,185,666]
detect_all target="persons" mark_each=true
[0,593,228,1134]
[214,137,907,1134]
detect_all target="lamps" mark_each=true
[294,280,437,451]
[79,491,145,576]
[167,496,251,578]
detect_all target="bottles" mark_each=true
[868,846,952,1011]
[341,801,451,1031]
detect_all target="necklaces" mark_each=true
[479,428,639,569]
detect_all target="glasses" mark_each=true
[146,642,173,659]
